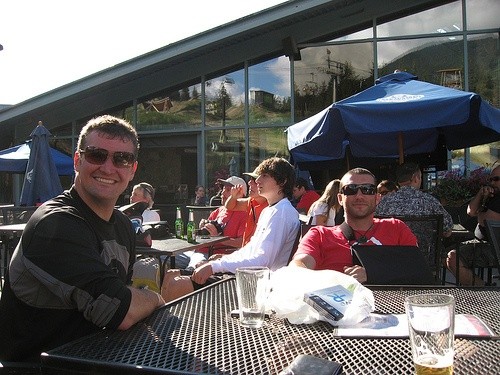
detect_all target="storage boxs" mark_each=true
[305,284,353,320]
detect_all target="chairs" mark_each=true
[373,212,444,286]
[470,219,500,285]
[0,205,38,291]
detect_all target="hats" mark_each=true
[243,166,260,178]
[218,176,247,197]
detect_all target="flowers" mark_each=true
[436,168,478,204]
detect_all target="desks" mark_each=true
[40,273,500,375]
[186,206,220,211]
[449,222,470,286]
[0,223,28,278]
[438,68,462,90]
[135,233,231,294]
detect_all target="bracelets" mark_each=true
[150,291,160,306]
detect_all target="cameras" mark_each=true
[195,220,223,235]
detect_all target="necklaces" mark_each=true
[353,223,374,243]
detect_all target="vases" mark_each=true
[441,200,463,212]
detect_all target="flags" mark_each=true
[327,49,331,54]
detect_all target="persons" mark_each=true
[190,185,206,206]
[441,165,500,286]
[228,157,239,176]
[292,176,321,214]
[196,176,255,253]
[374,163,454,261]
[160,157,300,304]
[130,183,154,246]
[306,179,341,226]
[209,167,268,260]
[0,114,169,375]
[289,168,418,283]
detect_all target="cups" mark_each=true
[404,293,455,375]
[234,266,270,328]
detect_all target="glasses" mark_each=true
[249,176,258,184]
[490,176,500,182]
[80,147,135,169]
[340,184,378,195]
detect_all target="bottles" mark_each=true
[175,207,184,239]
[187,209,196,243]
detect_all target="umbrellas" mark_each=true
[19,120,63,206]
[284,70,500,187]
[0,139,74,175]
